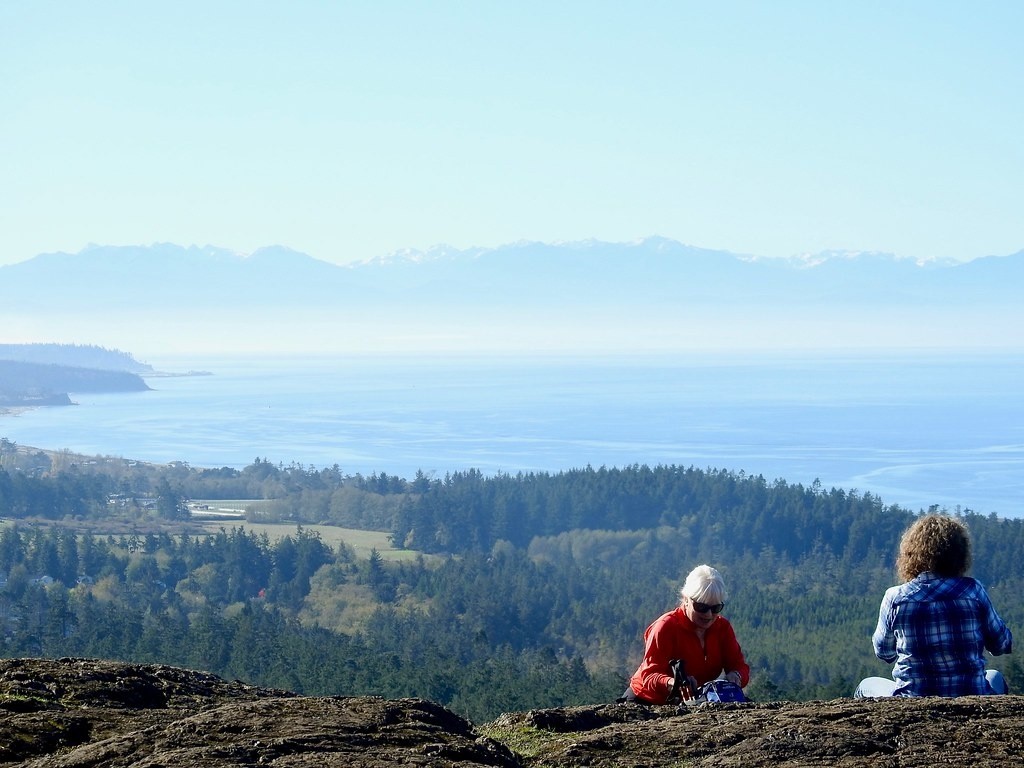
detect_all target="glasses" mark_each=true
[690,598,725,614]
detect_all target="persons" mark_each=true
[620,564,749,707]
[854,513,1012,698]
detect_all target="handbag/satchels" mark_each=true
[697,679,746,704]
